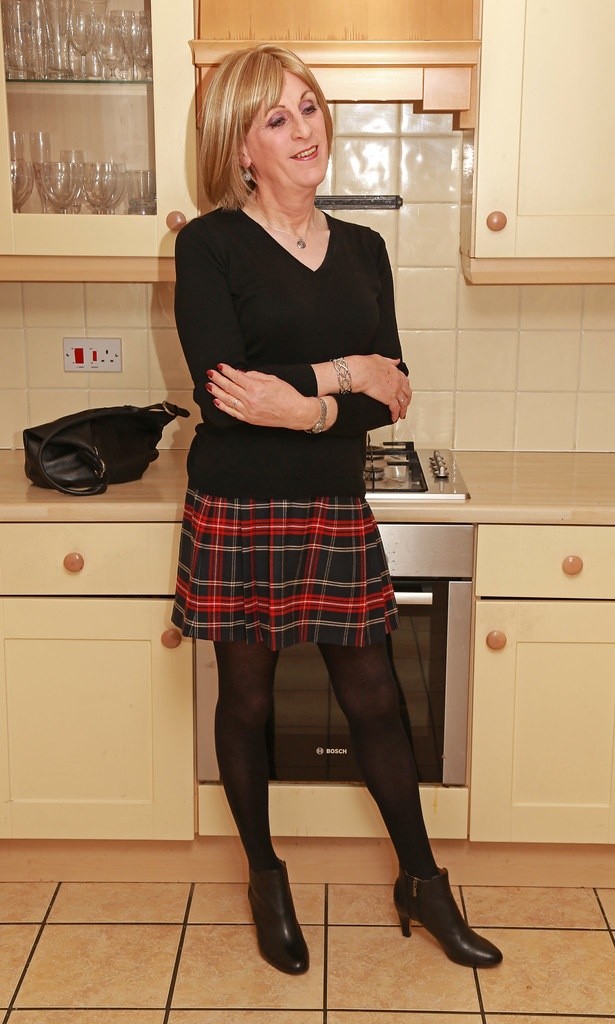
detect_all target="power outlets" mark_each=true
[63,337,122,373]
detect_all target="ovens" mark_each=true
[195,524,475,785]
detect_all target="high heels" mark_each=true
[248,857,309,975]
[393,862,503,967]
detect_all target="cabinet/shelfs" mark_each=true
[0,0,197,285]
[461,0,615,283]
[467,451,615,844]
[0,448,198,842]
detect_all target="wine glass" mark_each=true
[8,129,154,215]
[68,10,153,81]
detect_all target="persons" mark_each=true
[171,43,503,975]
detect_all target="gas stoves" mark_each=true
[363,432,471,501]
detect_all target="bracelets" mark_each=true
[306,398,327,434]
[332,357,352,395]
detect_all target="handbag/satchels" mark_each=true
[22,401,191,496]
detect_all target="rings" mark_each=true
[400,396,406,402]
[234,399,237,408]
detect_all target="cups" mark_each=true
[0,0,109,79]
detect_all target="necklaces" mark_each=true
[256,206,306,249]
